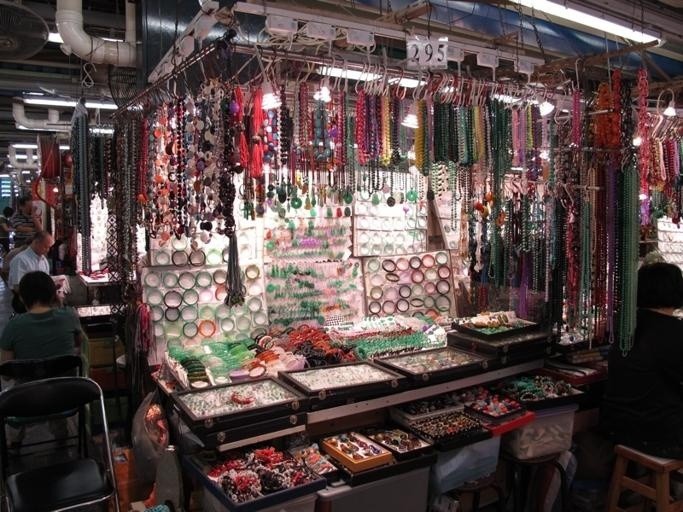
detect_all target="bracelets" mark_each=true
[180,360,267,390]
[497,374,574,402]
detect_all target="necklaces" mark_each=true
[69,68,682,359]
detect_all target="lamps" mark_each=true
[148,0,219,85]
[510,0,667,49]
[22,95,143,112]
[656,88,676,117]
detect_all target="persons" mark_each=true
[1,272,88,455]
[10,231,64,313]
[1,205,11,252]
[603,261,683,501]
[12,194,43,245]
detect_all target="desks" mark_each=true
[69,271,137,306]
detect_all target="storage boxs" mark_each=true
[197,479,317,512]
[431,434,501,494]
[577,431,611,469]
[316,464,431,512]
[508,403,578,460]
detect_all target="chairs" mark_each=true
[87,321,117,376]
[1,355,88,460]
[0,377,119,511]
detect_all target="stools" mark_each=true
[602,443,683,512]
[454,475,505,512]
[503,453,568,512]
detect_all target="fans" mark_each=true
[0,0,50,62]
